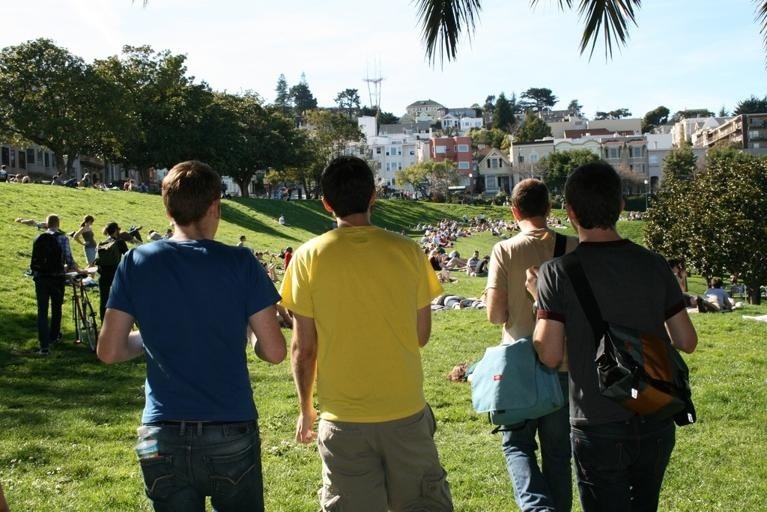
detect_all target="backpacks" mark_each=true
[98,238,119,266]
[30,233,65,277]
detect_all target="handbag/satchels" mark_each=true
[594,320,687,422]
[467,336,565,425]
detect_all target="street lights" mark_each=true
[644,178,649,208]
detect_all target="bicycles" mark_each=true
[70,272,98,352]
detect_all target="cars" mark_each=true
[389,192,404,200]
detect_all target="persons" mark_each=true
[279,215,291,227]
[96,221,173,328]
[376,183,418,200]
[669,258,744,312]
[619,212,648,221]
[221,190,233,200]
[0,164,153,193]
[417,210,520,313]
[98,161,286,511]
[524,163,699,511]
[384,225,413,236]
[488,178,580,512]
[546,213,571,230]
[276,157,452,511]
[275,303,292,328]
[28,214,292,354]
[236,235,292,281]
